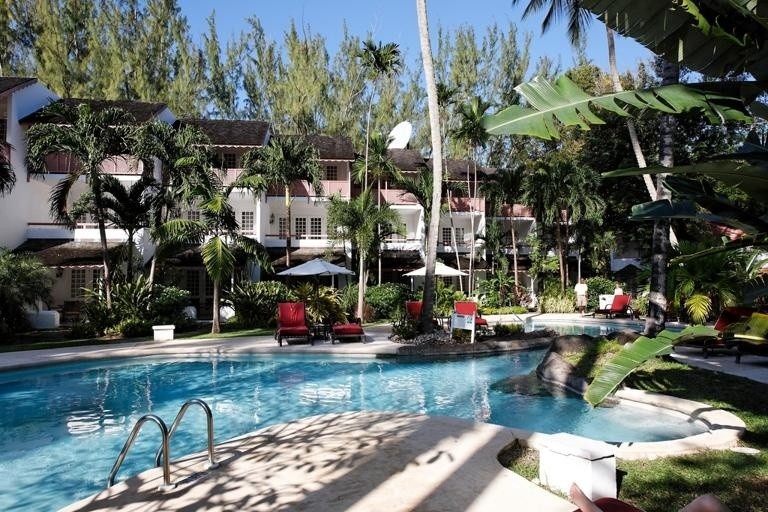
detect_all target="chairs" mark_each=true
[274,301,312,346]
[595,293,634,319]
[406,301,425,318]
[329,316,364,341]
[570,483,730,512]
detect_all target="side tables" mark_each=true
[308,322,328,337]
[433,313,450,328]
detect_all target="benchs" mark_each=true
[703,306,755,356]
[453,299,488,335]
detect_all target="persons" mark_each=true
[573,278,588,317]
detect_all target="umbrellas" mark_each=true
[278,257,355,323]
[403,262,470,310]
